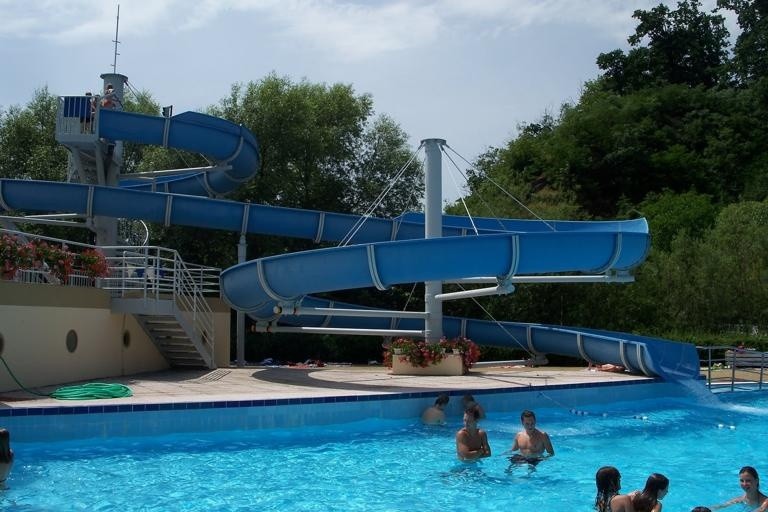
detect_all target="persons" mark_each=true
[592,466,633,512]
[421,395,450,424]
[628,473,669,512]
[80,84,120,134]
[455,408,491,460]
[506,409,554,478]
[462,394,485,419]
[710,466,768,512]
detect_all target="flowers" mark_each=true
[78,247,111,287]
[36,239,77,285]
[1,234,43,280]
[379,336,480,375]
